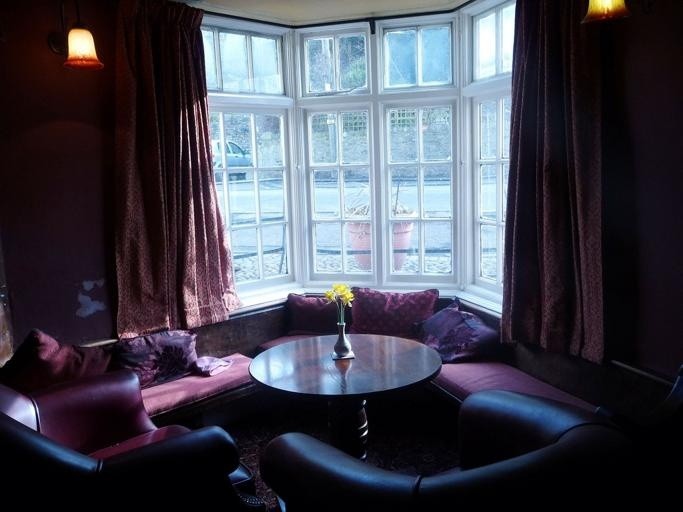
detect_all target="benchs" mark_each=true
[66,288,675,454]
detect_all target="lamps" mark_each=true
[580,0,656,25]
[45,27,106,73]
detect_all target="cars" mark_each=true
[212,141,251,182]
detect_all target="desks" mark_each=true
[246,332,442,462]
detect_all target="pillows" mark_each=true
[2,324,200,390]
[284,286,500,365]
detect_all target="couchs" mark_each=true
[3,373,254,510]
[258,388,624,511]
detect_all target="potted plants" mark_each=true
[337,179,419,274]
